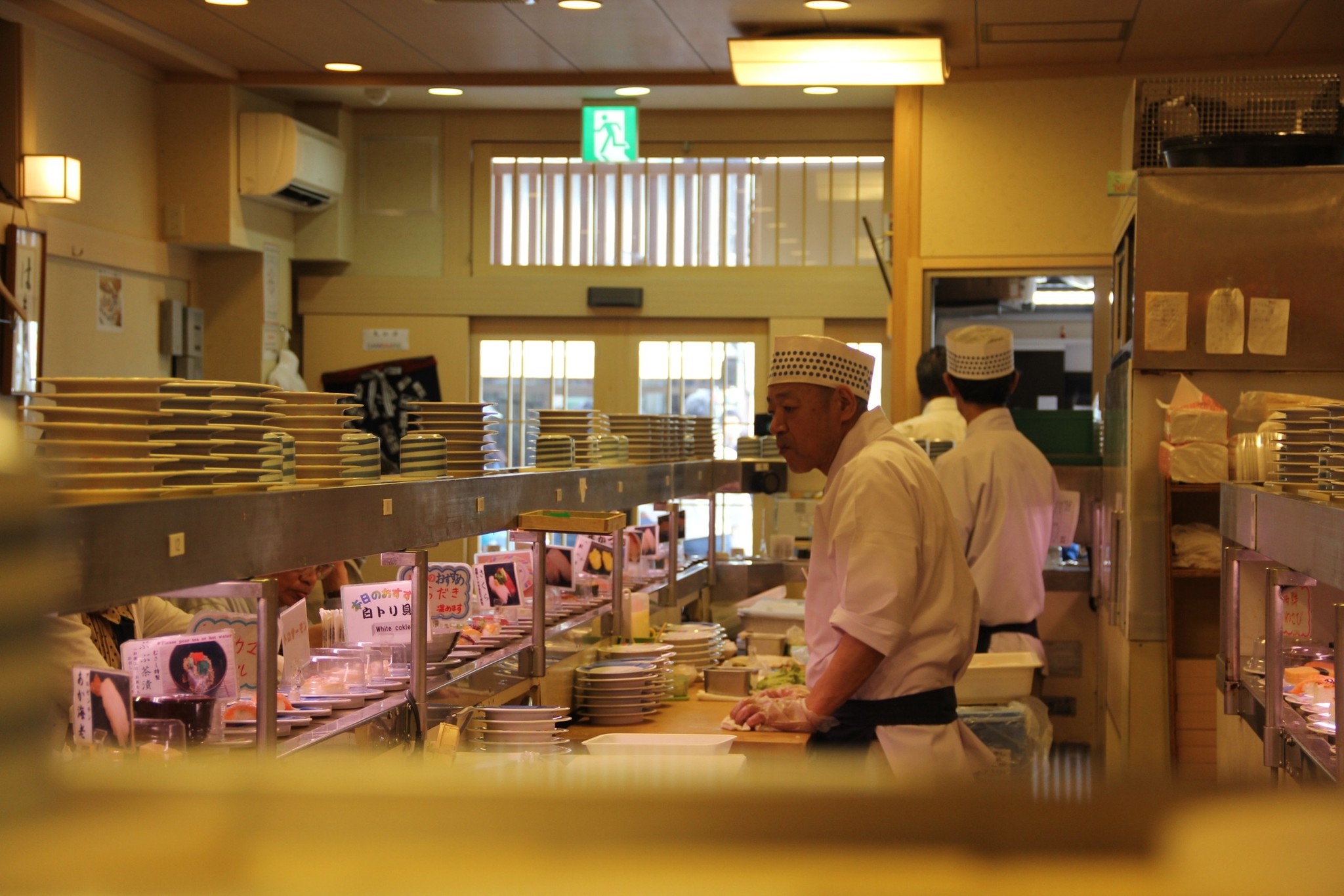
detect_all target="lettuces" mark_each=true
[754,663,806,691]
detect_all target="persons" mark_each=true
[729,334,978,755]
[177,561,350,647]
[44,595,193,751]
[893,345,967,447]
[934,325,1059,700]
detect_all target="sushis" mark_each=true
[451,616,507,646]
[587,548,613,576]
[627,528,656,562]
[224,662,387,721]
[489,568,516,605]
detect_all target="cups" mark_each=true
[535,434,573,467]
[571,438,576,467]
[258,434,282,483]
[929,440,953,466]
[279,434,296,485]
[400,433,449,478]
[617,435,630,463]
[909,439,925,451]
[593,434,620,465]
[1317,445,1331,489]
[340,433,383,480]
[737,437,785,459]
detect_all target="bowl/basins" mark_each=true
[168,642,229,694]
[131,695,222,744]
[404,629,465,661]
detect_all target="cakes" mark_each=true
[1283,662,1335,701]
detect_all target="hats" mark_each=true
[945,325,1015,380]
[766,334,876,404]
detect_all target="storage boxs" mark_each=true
[1175,657,1218,786]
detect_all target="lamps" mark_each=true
[722,29,958,94]
[17,152,85,209]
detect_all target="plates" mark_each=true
[447,558,698,658]
[425,701,465,726]
[1264,403,1344,501]
[15,376,717,504]
[225,658,460,738]
[581,733,737,757]
[1243,648,1336,738]
[573,619,727,723]
[476,706,574,757]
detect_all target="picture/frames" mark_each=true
[0,223,50,399]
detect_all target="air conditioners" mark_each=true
[235,107,350,215]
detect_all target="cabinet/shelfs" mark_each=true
[1159,476,1222,785]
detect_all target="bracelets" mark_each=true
[323,590,341,599]
[801,698,829,721]
